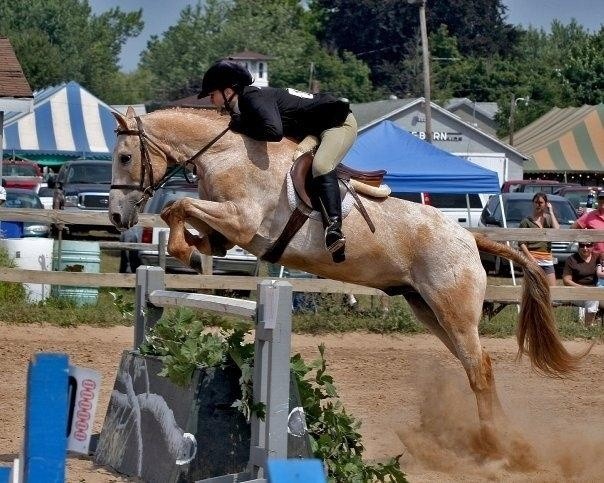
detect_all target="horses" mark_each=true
[107,106,597,456]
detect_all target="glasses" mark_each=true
[579,243,593,249]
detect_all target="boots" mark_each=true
[312,169,346,263]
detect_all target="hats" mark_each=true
[597,190,604,198]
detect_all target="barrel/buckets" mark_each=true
[1,238,30,301]
[24,236,55,305]
[52,239,107,311]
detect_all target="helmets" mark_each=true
[196,60,253,99]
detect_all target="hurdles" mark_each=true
[94,265,324,482]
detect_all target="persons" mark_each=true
[194,59,359,264]
[569,191,603,266]
[516,190,561,286]
[561,241,603,325]
